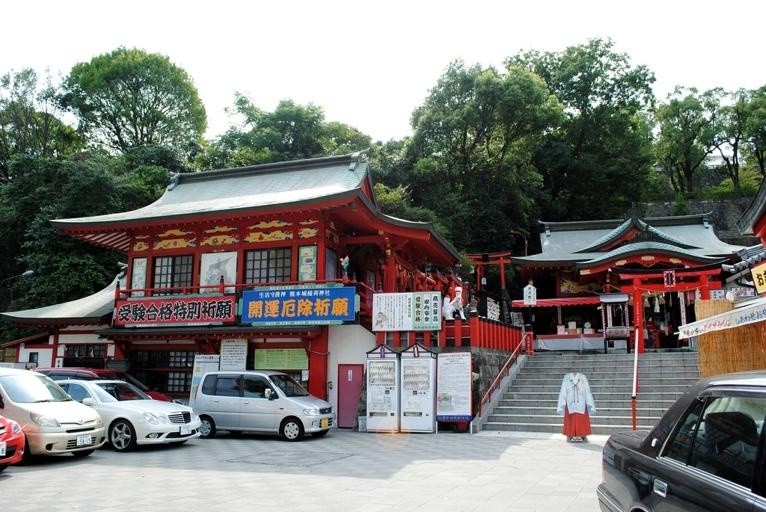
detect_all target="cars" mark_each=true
[596,369,766,512]
[0,362,204,472]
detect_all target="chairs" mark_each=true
[695,411,759,489]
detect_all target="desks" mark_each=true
[536,333,606,355]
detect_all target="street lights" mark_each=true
[510,229,529,257]
[0,270,34,283]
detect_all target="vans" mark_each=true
[194,369,335,442]
